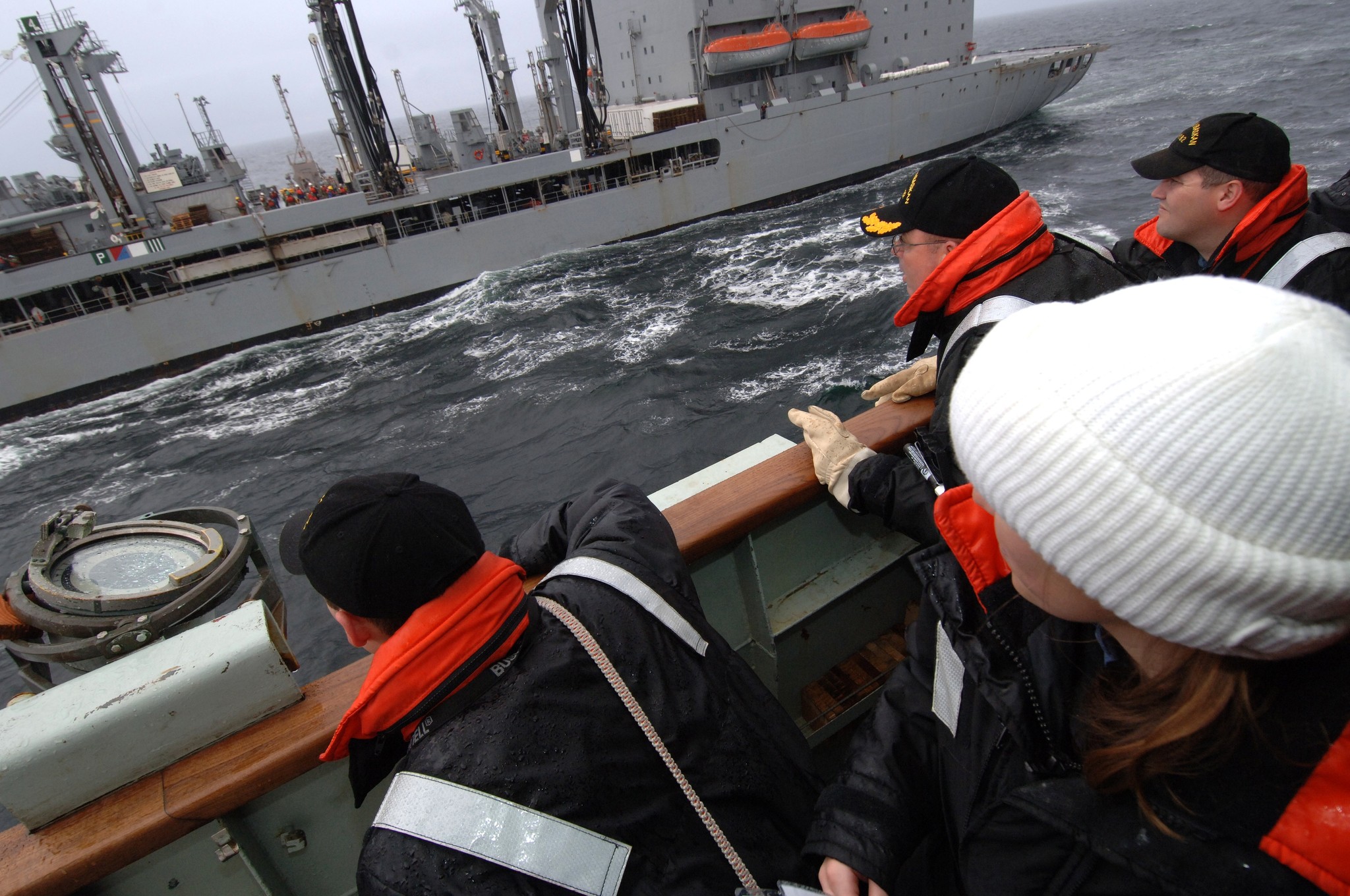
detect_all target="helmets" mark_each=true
[260,168,342,202]
[235,197,240,200]
[763,102,765,104]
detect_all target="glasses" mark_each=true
[891,232,947,255]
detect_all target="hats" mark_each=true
[860,155,1020,240]
[947,275,1350,662]
[1130,112,1290,182]
[280,475,485,618]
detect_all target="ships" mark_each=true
[0,0,1101,427]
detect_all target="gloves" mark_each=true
[861,355,937,407]
[788,405,878,516]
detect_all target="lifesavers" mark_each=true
[474,151,483,161]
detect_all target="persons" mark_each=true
[785,156,1135,545]
[103,116,710,247]
[282,470,846,896]
[812,270,1350,896]
[1113,111,1350,314]
